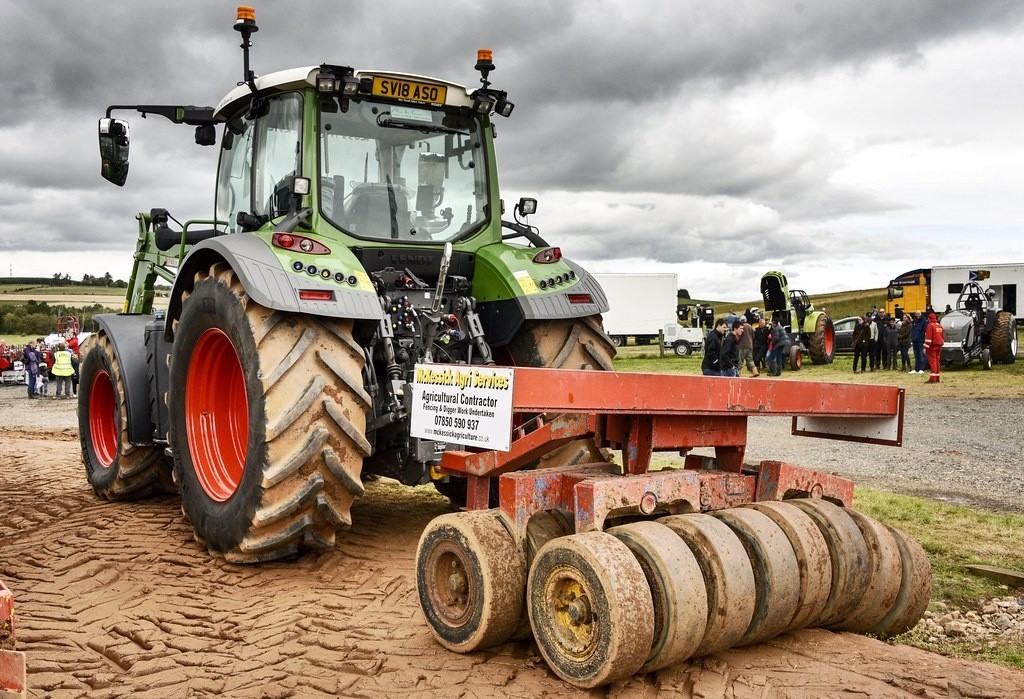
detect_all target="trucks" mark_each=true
[591,272,678,347]
[883,262,1024,320]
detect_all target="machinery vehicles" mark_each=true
[940,281,1019,370]
[750,271,835,372]
[77,4,621,567]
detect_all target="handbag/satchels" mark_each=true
[21,357,30,365]
[851,340,857,348]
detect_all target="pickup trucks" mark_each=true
[831,315,861,353]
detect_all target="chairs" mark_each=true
[348,183,411,232]
[319,175,346,220]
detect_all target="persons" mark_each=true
[945,305,952,314]
[852,310,927,374]
[895,304,904,321]
[66,332,81,356]
[692,304,700,327]
[872,305,878,316]
[724,309,738,328]
[701,319,728,377]
[719,321,745,377]
[737,316,791,377]
[926,305,934,314]
[923,313,944,383]
[24,337,79,400]
[879,309,885,317]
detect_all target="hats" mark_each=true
[772,317,778,324]
[739,316,747,322]
[928,313,937,322]
[866,312,872,317]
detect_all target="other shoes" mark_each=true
[910,365,912,371]
[885,367,890,370]
[861,369,866,373]
[876,365,881,369]
[28,391,77,400]
[894,367,899,370]
[871,367,874,371]
[853,370,857,373]
[768,371,780,376]
[899,367,906,371]
[917,370,924,374]
[908,370,917,374]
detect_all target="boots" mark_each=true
[936,374,939,382]
[929,374,936,383]
[752,367,759,377]
